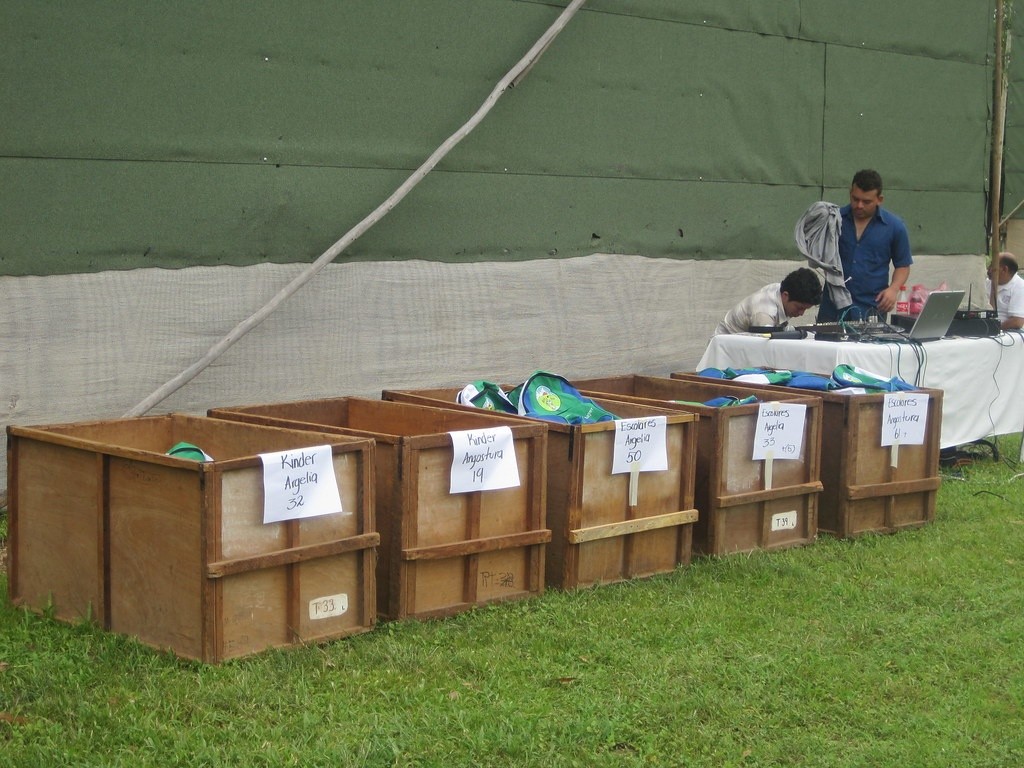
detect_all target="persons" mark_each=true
[710,267,822,334]
[986,250,1024,331]
[795,169,913,322]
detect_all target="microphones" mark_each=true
[770,329,808,340]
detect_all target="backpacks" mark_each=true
[161,442,213,465]
[672,364,915,409]
[455,371,623,430]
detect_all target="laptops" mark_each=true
[871,291,966,342]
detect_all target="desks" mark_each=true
[695,331,1024,462]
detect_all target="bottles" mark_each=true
[910,285,922,314]
[896,285,908,315]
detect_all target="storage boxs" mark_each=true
[208,394,548,621]
[383,383,700,592]
[6,414,381,665]
[671,366,942,543]
[571,364,824,559]
